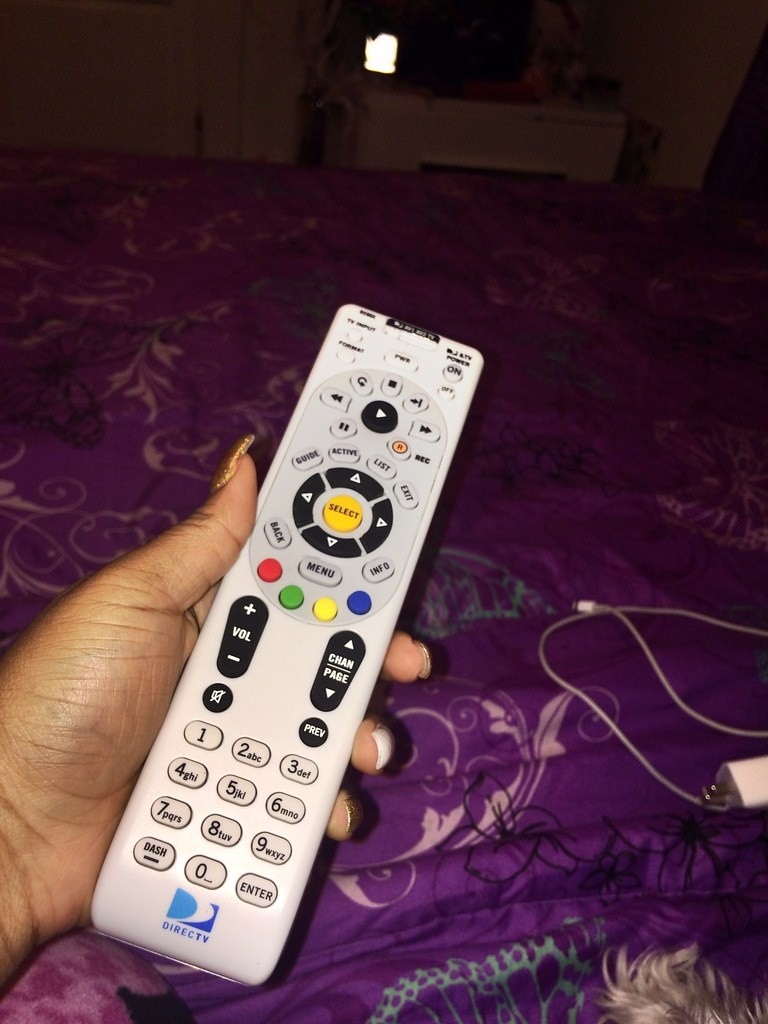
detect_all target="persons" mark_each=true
[1,432,431,991]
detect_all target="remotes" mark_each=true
[91,302,486,986]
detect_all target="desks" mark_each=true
[324,82,626,181]
